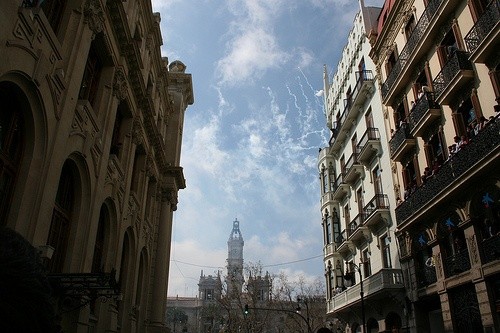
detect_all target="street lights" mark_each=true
[342,261,366,333]
[294,297,310,333]
[243,304,312,333]
